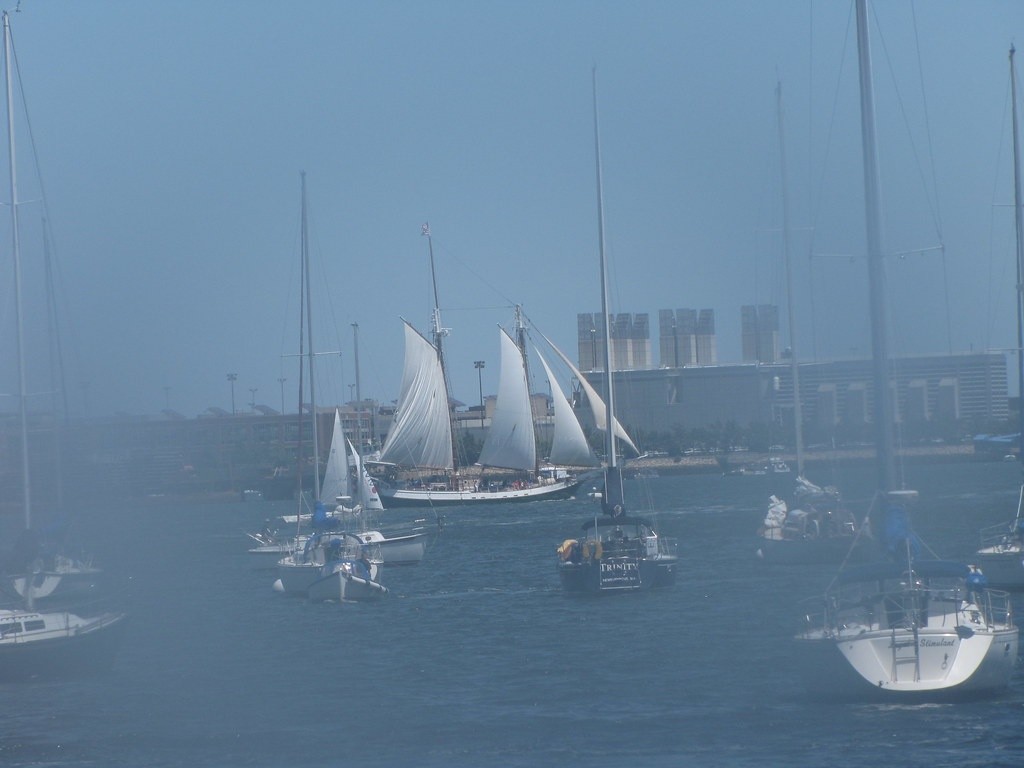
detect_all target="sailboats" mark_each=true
[966,43,1023,591]
[753,62,875,558]
[356,221,647,506]
[555,66,682,595]
[2,9,132,673]
[245,166,448,606]
[789,1,1020,705]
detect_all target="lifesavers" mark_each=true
[583,540,603,560]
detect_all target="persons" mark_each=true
[407,477,414,489]
[502,478,528,490]
